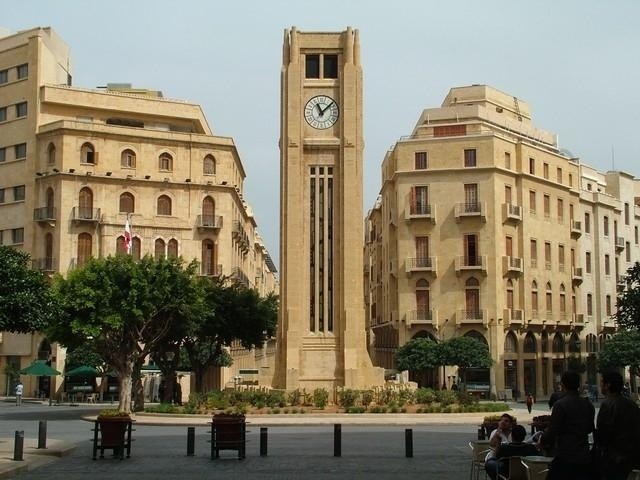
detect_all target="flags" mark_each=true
[121,219,132,251]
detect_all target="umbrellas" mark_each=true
[19,360,61,376]
[64,366,103,377]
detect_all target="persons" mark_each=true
[14,382,24,406]
[485,370,640,480]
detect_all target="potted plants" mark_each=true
[211,407,247,448]
[97,407,130,447]
[481,416,555,433]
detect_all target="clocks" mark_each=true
[302,96,340,131]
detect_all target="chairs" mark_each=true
[465,434,556,478]
[59,389,100,404]
